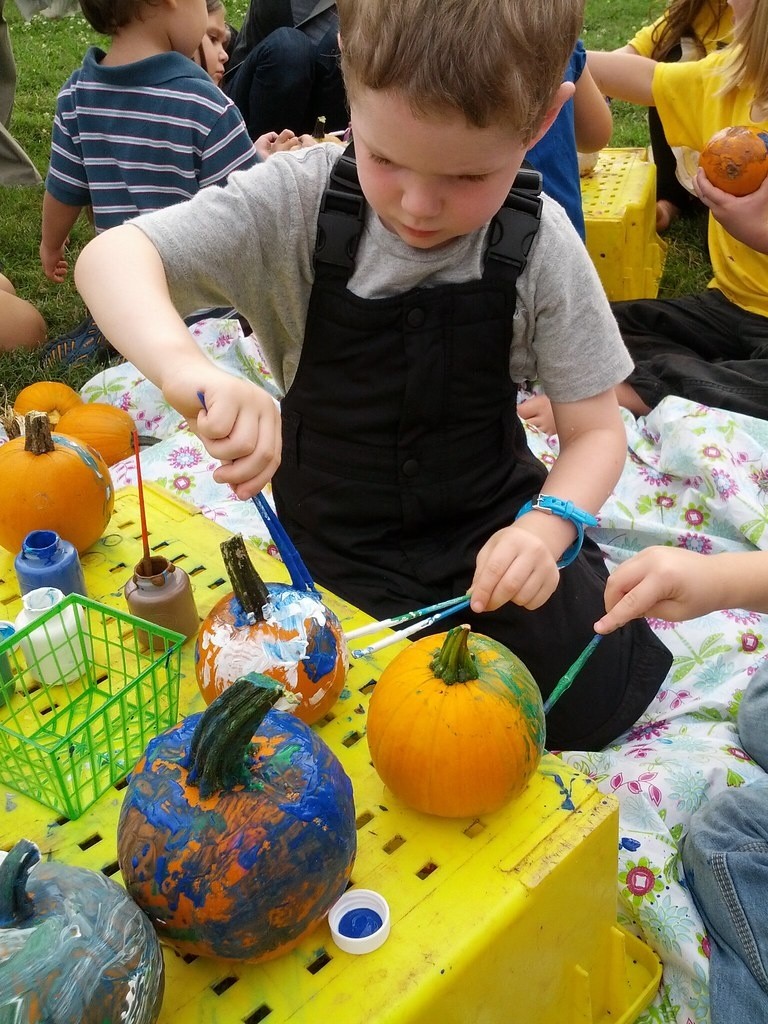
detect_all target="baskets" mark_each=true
[0,592,189,822]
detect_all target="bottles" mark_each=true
[14,529,87,609]
[124,556,200,649]
[15,586,93,685]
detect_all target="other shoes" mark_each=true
[39,314,110,374]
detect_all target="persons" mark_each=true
[0,273,46,351]
[39,0,301,282]
[524,39,612,245]
[516,0,768,434]
[219,0,351,138]
[73,0,674,752]
[594,545,768,1024]
[611,0,735,233]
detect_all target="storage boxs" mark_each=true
[577,146,672,302]
[0,484,664,1024]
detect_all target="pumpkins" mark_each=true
[12,382,84,434]
[308,116,342,144]
[0,409,115,557]
[194,532,349,726]
[116,673,358,966]
[698,125,768,196]
[367,623,546,820]
[0,838,165,1024]
[54,403,162,468]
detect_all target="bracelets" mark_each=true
[514,493,597,568]
